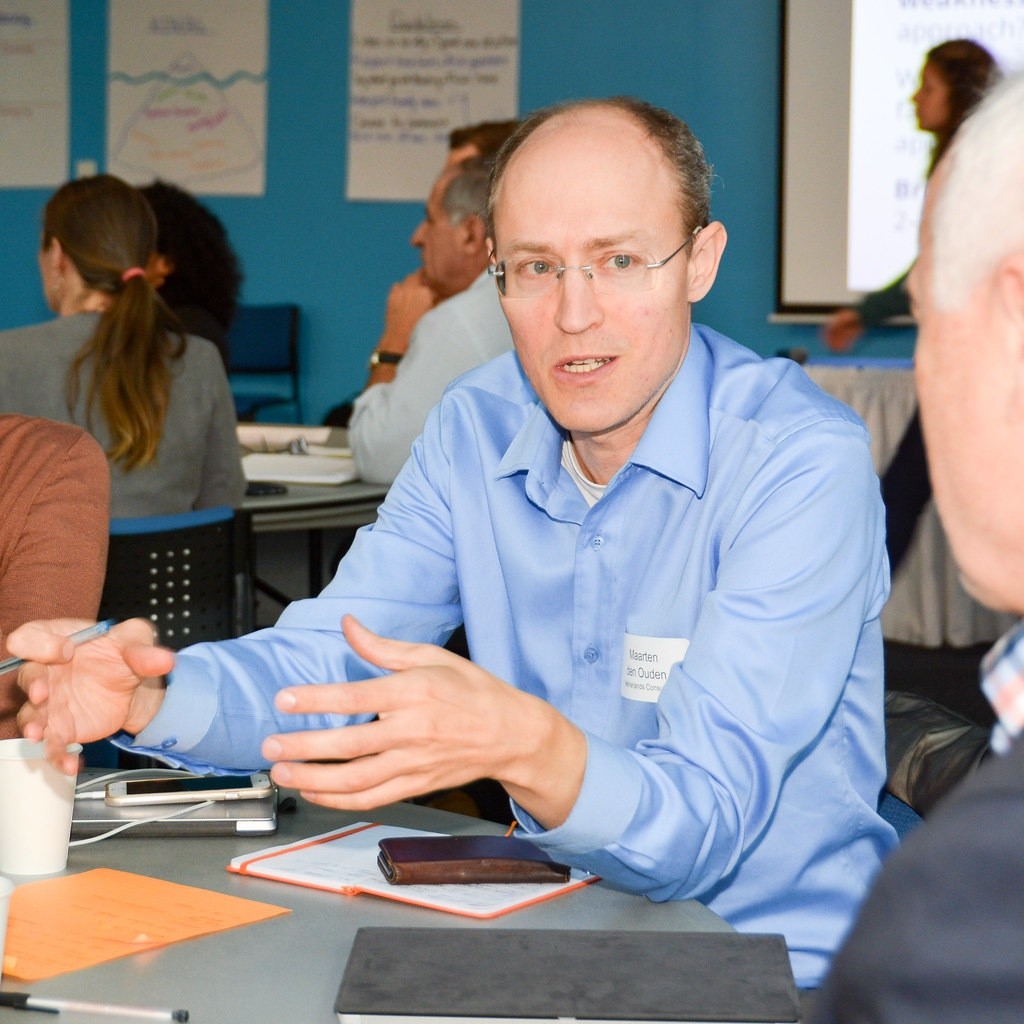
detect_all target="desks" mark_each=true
[800,363,1022,649]
[240,476,393,610]
[1,768,735,1024]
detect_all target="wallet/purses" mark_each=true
[378,835,571,885]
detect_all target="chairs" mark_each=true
[225,304,305,424]
[81,505,241,767]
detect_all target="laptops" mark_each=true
[72,773,279,835]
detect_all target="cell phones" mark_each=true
[105,773,276,806]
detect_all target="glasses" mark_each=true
[487,225,704,298]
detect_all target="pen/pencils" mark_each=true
[0,992,189,1023]
[0,621,111,676]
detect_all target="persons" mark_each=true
[7,97,899,1009]
[1,116,540,751]
[808,38,1023,1024]
